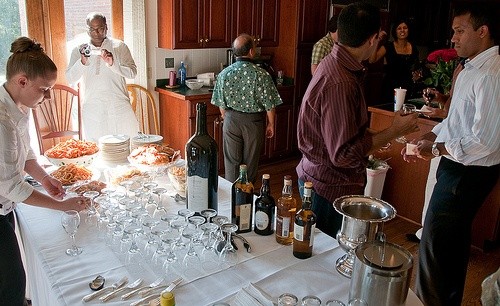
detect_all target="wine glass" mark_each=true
[423,88,437,118]
[83,177,239,275]
[394,104,417,143]
[61,209,84,257]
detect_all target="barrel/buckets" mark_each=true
[349,240,414,306]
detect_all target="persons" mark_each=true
[400,5,500,305]
[311,14,338,75]
[67,12,137,139]
[370,21,422,103]
[0,36,93,306]
[210,34,284,185]
[296,3,420,237]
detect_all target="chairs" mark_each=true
[32,83,83,156]
[126,84,159,136]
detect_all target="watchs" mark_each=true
[431,142,440,157]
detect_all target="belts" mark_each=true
[228,107,264,114]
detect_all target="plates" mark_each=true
[61,179,91,190]
[99,134,164,163]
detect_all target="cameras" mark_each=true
[84,44,103,57]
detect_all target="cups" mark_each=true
[169,71,177,86]
[277,294,368,306]
[393,88,407,112]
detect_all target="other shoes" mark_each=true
[405,233,420,243]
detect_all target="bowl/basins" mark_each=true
[127,156,187,192]
[185,79,204,90]
[44,148,99,166]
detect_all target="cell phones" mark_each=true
[209,85,214,91]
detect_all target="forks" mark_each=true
[82,275,184,306]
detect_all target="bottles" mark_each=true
[254,173,275,235]
[185,102,217,222]
[293,182,317,259]
[177,61,186,85]
[160,291,175,306]
[276,174,297,246]
[231,164,254,233]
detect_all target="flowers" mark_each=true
[425,48,458,94]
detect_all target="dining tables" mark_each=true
[13,144,423,306]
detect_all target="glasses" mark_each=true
[86,25,106,34]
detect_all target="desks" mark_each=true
[367,97,500,250]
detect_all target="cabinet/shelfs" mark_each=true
[282,0,330,43]
[229,0,279,47]
[154,88,293,173]
[158,0,231,50]
